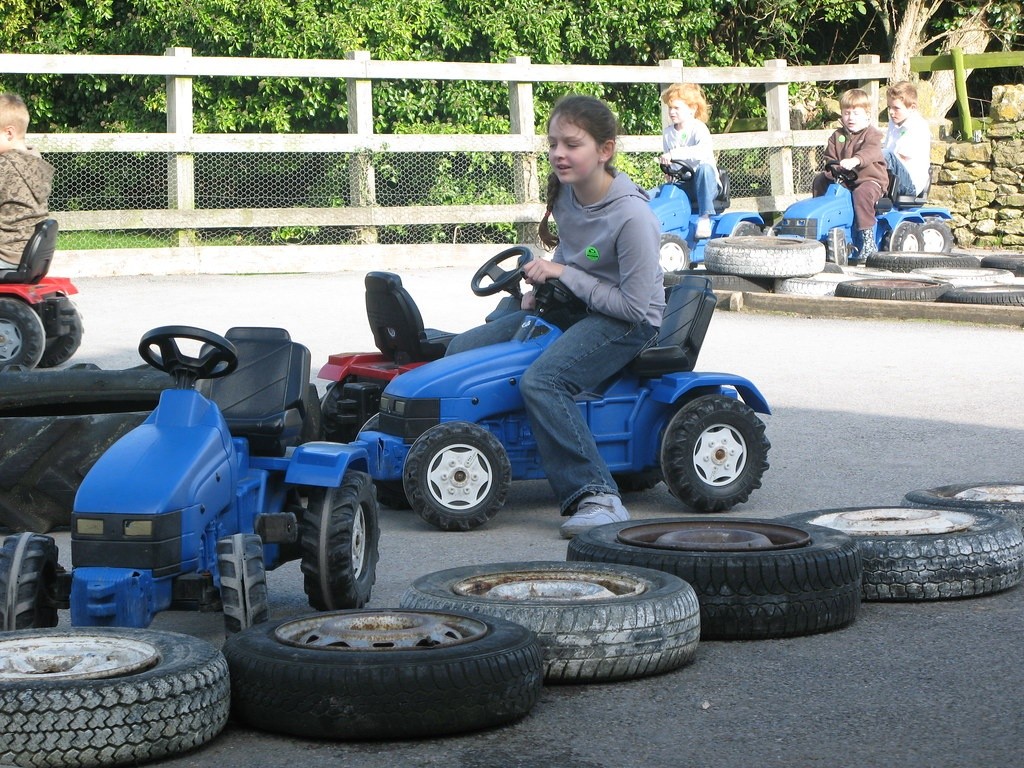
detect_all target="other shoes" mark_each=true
[695,218,711,238]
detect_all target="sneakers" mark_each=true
[559,490,630,539]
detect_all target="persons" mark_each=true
[655,84,929,264]
[0,95,55,274]
[426,96,669,538]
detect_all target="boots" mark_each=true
[858,228,878,264]
